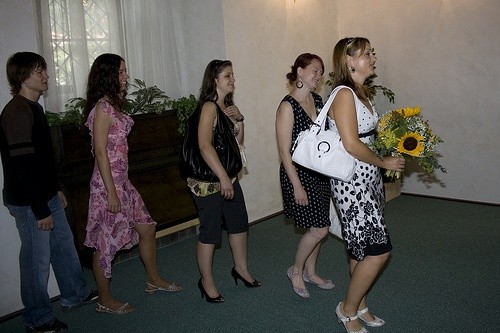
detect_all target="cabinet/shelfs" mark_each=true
[47,100,201,251]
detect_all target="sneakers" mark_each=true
[62,289,99,313]
[25,317,68,333]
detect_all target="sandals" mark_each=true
[303,271,335,289]
[145,280,183,295]
[286,265,309,299]
[96,303,134,316]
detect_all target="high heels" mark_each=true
[356,306,385,327]
[335,302,369,333]
[198,277,225,303]
[231,267,263,288]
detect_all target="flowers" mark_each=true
[375,107,447,178]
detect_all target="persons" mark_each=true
[275,53,336,298]
[0,52,99,333]
[185,59,264,303]
[328,37,405,333]
[79,54,182,314]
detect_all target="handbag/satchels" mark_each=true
[184,99,242,182]
[329,197,343,240]
[291,85,356,183]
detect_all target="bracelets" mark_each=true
[236,118,244,122]
